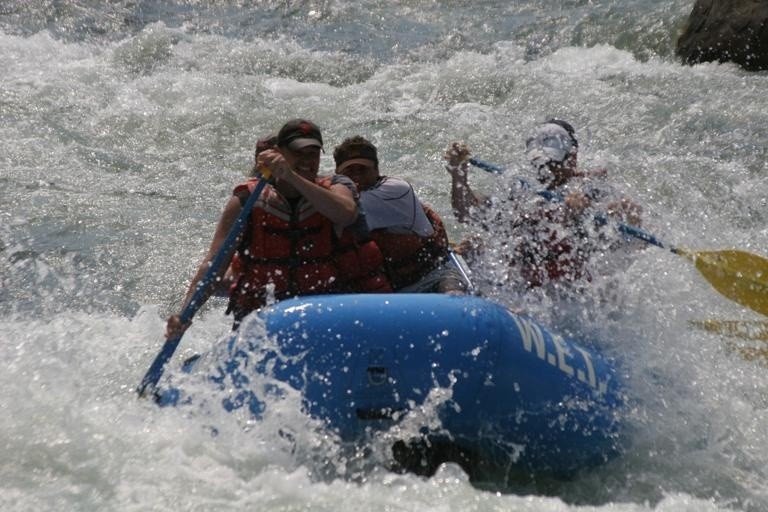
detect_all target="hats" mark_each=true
[256,119,325,152]
[527,119,578,168]
[334,135,377,173]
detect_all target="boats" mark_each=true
[159,269,707,489]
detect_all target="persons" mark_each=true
[332,135,475,300]
[218,134,283,297]
[441,117,647,342]
[164,118,395,354]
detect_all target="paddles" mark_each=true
[460,141,768,318]
